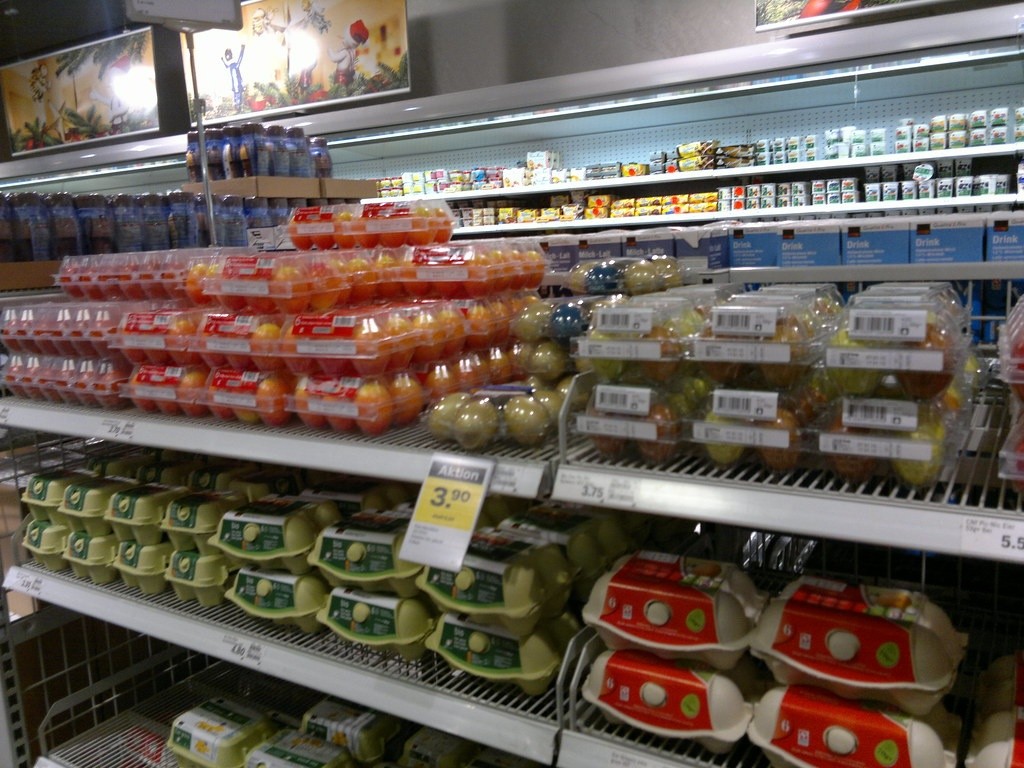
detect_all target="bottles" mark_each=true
[187,123,333,182]
[0,191,361,262]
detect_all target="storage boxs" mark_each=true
[447,209,1024,267]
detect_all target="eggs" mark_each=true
[0,207,971,482]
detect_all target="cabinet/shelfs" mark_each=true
[0,399,1024,768]
[364,141,1024,280]
